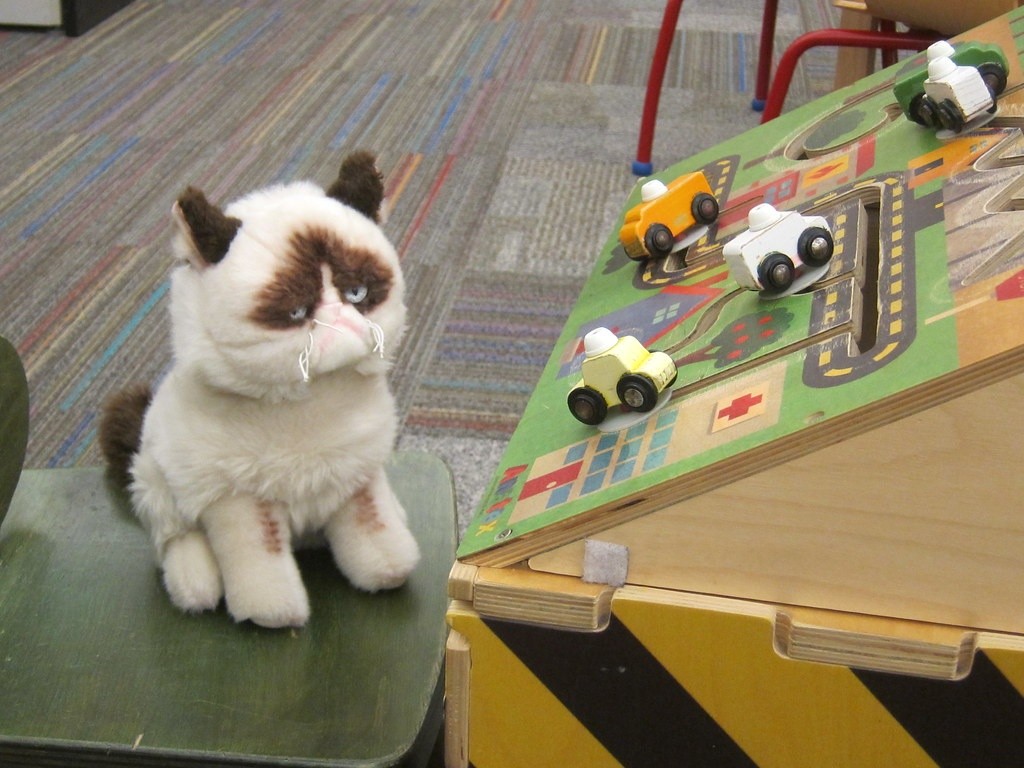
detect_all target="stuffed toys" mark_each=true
[100,153,418,630]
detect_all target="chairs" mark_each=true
[0,335,460,768]
